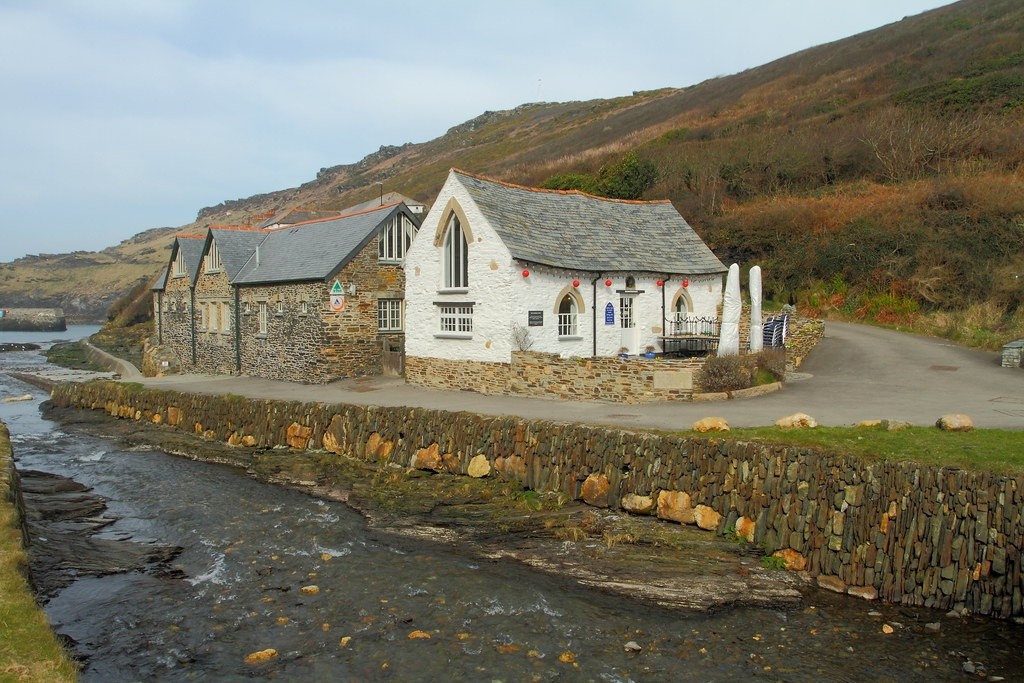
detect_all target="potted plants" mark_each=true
[618,347,629,358]
[645,346,656,359]
